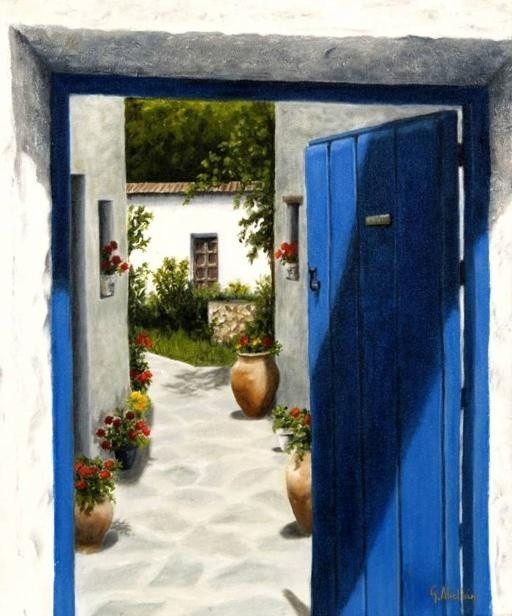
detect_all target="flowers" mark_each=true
[100,241,129,274]
[74,331,155,511]
[234,331,284,357]
[286,403,312,462]
[275,239,298,265]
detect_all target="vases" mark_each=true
[229,349,280,417]
[74,497,113,554]
[100,273,117,297]
[284,261,299,281]
[285,446,313,534]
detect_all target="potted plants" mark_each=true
[274,406,297,451]
